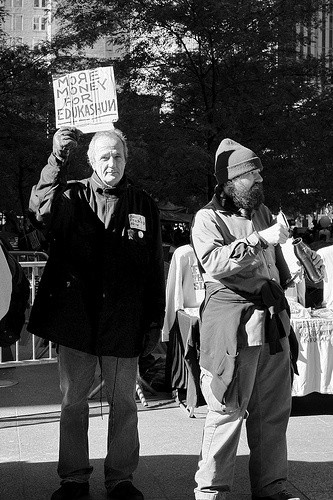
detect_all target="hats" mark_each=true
[213,137,263,185]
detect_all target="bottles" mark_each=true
[292,238,324,284]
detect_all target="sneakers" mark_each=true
[51,479,90,500]
[107,481,145,500]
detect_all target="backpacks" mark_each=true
[0,242,30,347]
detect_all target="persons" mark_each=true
[28,126,165,500]
[190,139,325,500]
